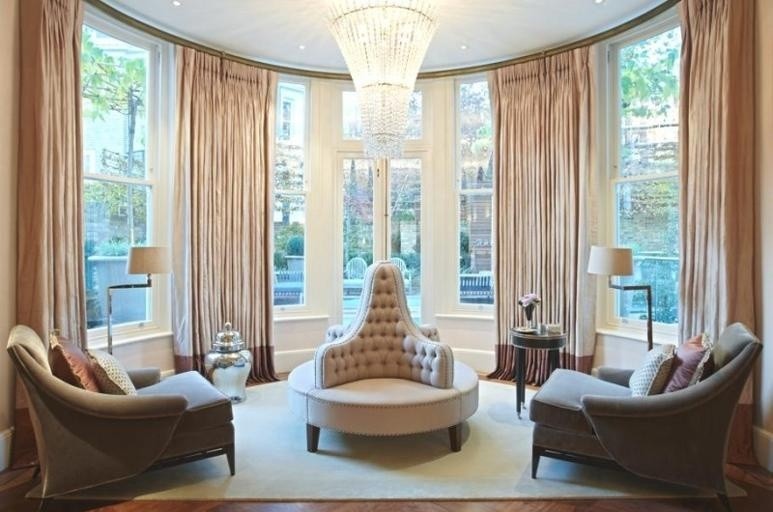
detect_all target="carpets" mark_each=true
[25,373,751,501]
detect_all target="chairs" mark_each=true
[6,326,236,512]
[287,262,480,454]
[530,322,766,512]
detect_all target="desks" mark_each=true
[510,328,567,419]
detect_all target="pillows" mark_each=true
[664,332,712,393]
[628,343,675,396]
[84,347,138,395]
[48,329,103,393]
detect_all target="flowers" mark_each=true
[518,293,542,321]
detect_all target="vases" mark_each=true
[204,321,254,405]
[525,321,531,331]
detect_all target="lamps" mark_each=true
[319,0,440,162]
[586,245,654,351]
[105,247,170,351]
